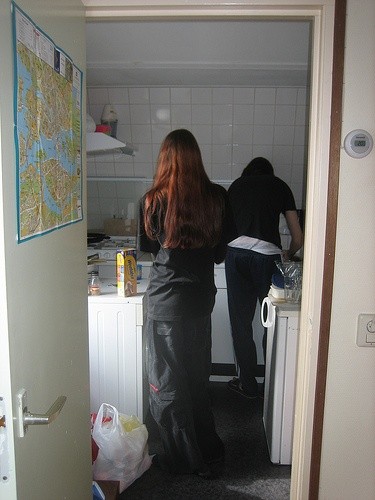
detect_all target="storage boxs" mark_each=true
[117,248,137,297]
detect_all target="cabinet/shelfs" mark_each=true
[86,254,264,427]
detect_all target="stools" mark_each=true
[93,480,119,500]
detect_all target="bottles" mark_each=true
[88,271,101,296]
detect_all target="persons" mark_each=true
[224,157,304,400]
[135,128,234,477]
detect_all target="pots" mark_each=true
[86,232,109,243]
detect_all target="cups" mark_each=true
[284,263,302,304]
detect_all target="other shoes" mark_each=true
[152,452,171,476]
[227,379,264,399]
